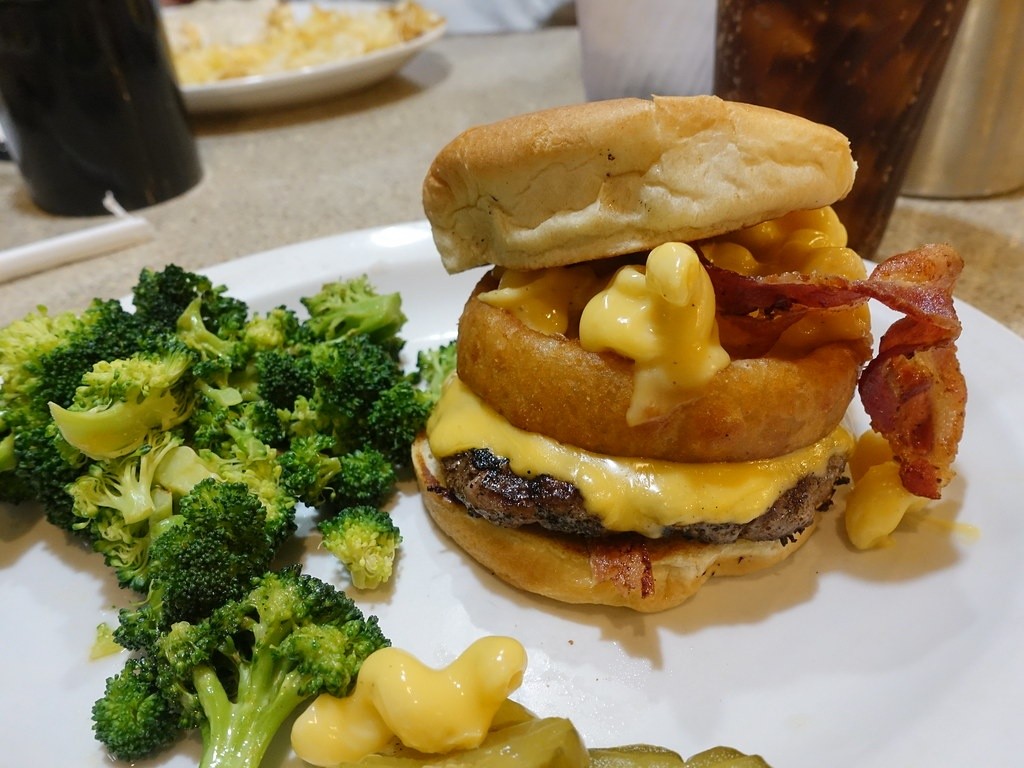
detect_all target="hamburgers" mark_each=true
[409,95,965,613]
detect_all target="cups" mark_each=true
[0,0,202,219]
[717,0,968,259]
[879,0,1024,202]
[575,0,717,102]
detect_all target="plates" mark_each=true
[179,9,446,110]
[0,220,1024,768]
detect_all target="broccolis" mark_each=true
[0,263,457,767]
